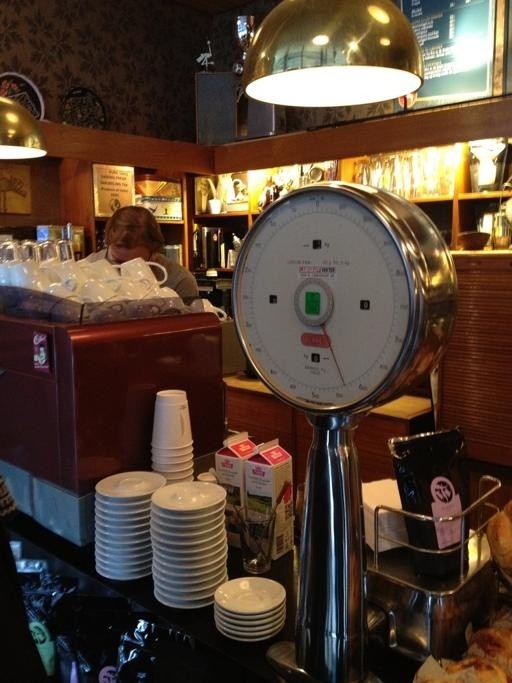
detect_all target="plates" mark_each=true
[93,471,166,581]
[150,481,228,609]
[213,575,287,643]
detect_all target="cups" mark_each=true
[14,258,48,291]
[79,278,113,309]
[86,257,119,282]
[119,257,168,288]
[113,277,149,303]
[150,390,194,483]
[176,475,194,481]
[73,259,84,266]
[60,258,78,286]
[49,283,78,304]
[0,256,18,288]
[41,256,67,288]
[154,287,178,297]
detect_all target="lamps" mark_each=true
[0,96,47,159]
[242,2,424,108]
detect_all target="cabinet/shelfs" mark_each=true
[0,121,214,304]
[224,374,432,491]
[215,96,512,316]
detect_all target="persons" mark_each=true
[79,205,199,307]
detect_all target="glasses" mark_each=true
[104,248,153,265]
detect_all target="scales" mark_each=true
[231,181,500,683]
